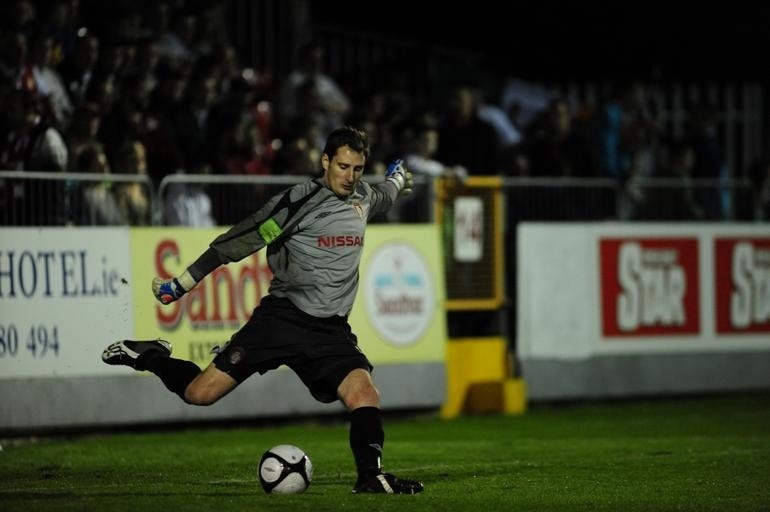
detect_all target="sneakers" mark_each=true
[350,471,425,495]
[101,340,172,371]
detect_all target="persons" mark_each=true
[100,128,428,496]
[1,4,769,228]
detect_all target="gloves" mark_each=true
[383,159,414,198]
[151,275,185,306]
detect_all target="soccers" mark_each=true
[258,444,313,495]
